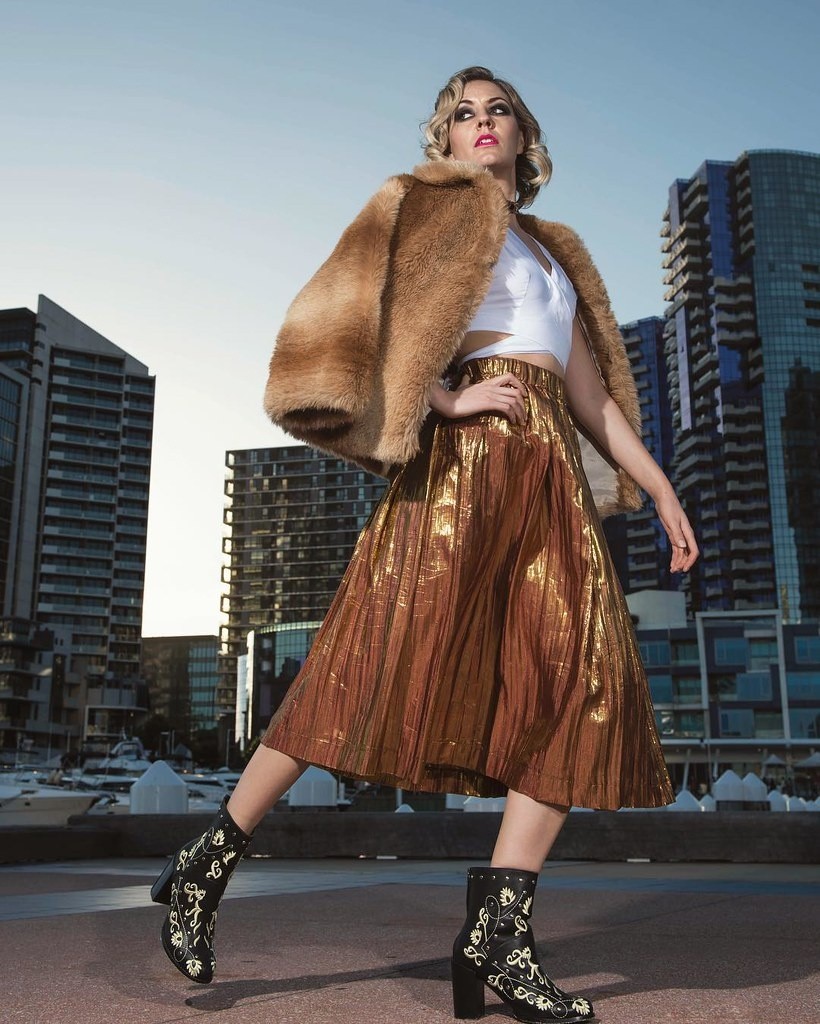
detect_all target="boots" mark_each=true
[149,796,256,984]
[448,867,592,1023]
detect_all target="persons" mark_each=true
[47,767,66,785]
[148,65,702,1024]
